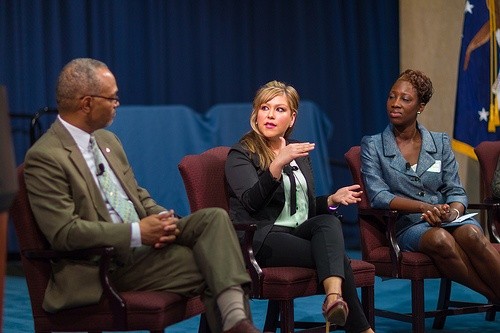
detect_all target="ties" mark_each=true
[89,135,141,224]
[282,164,299,217]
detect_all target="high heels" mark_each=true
[322,293,349,333]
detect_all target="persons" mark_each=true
[223,81,378,333]
[360,67,500,311]
[22,57,263,333]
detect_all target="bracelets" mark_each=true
[453,208,459,221]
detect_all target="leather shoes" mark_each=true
[222,317,260,333]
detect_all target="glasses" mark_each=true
[80,93,121,102]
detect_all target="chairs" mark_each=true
[346,146,499,333]
[475,142,500,209]
[178,147,376,333]
[15,164,202,333]
